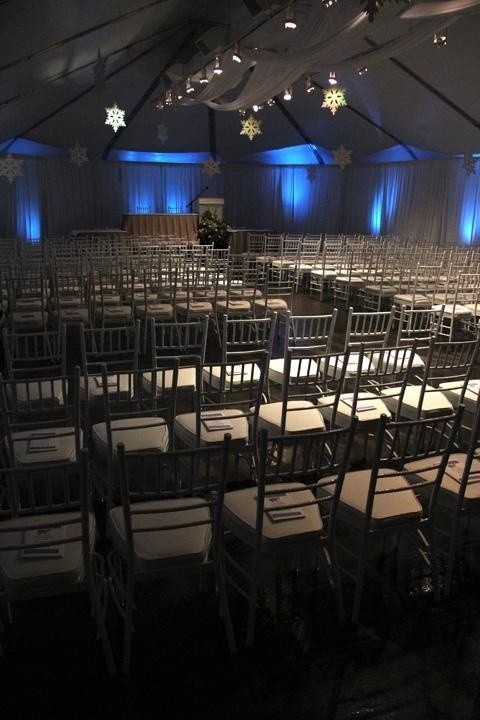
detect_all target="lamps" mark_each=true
[238,30,451,118]
[148,0,342,111]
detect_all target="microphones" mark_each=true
[204,186,209,192]
